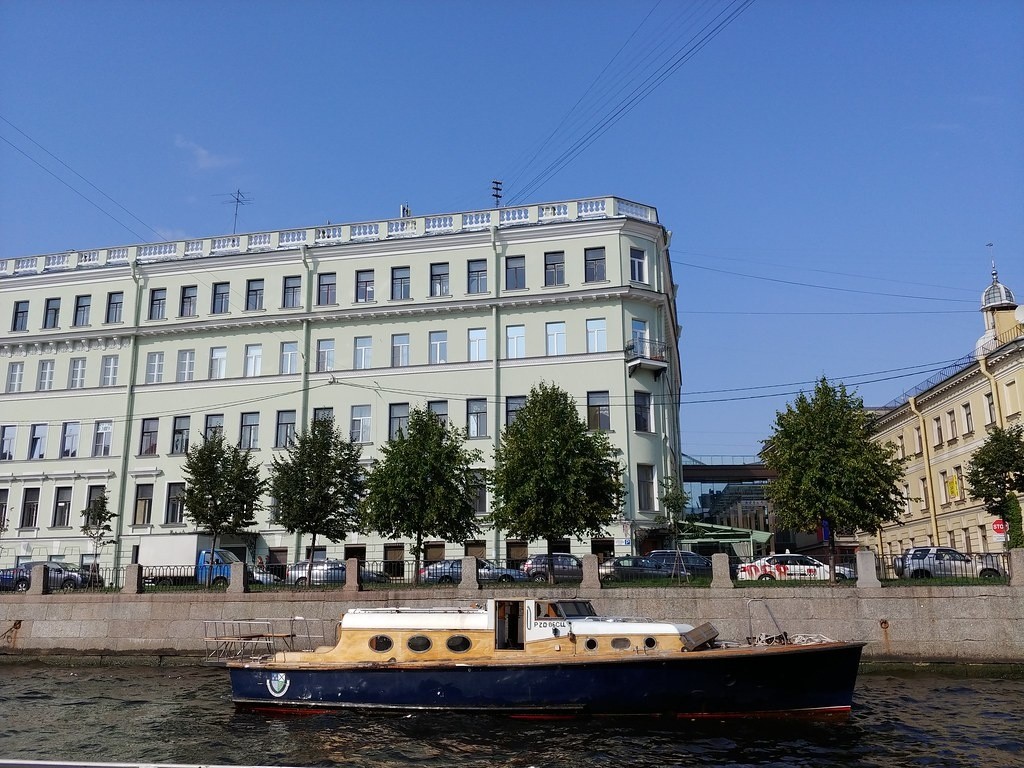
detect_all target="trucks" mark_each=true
[137,533,242,586]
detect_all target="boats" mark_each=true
[227,596,865,732]
[197,618,343,667]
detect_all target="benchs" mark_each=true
[205,633,297,658]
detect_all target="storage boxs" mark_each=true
[679,621,720,651]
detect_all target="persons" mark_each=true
[265,555,271,572]
[256,556,265,572]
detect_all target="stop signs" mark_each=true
[992,519,1009,534]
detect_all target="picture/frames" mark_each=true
[266,672,291,698]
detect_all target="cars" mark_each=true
[418,556,529,583]
[737,554,855,582]
[599,554,692,582]
[253,567,280,587]
[287,557,389,588]
[0,568,19,591]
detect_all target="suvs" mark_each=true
[525,552,584,583]
[644,550,738,581]
[894,546,1003,579]
[19,561,104,594]
